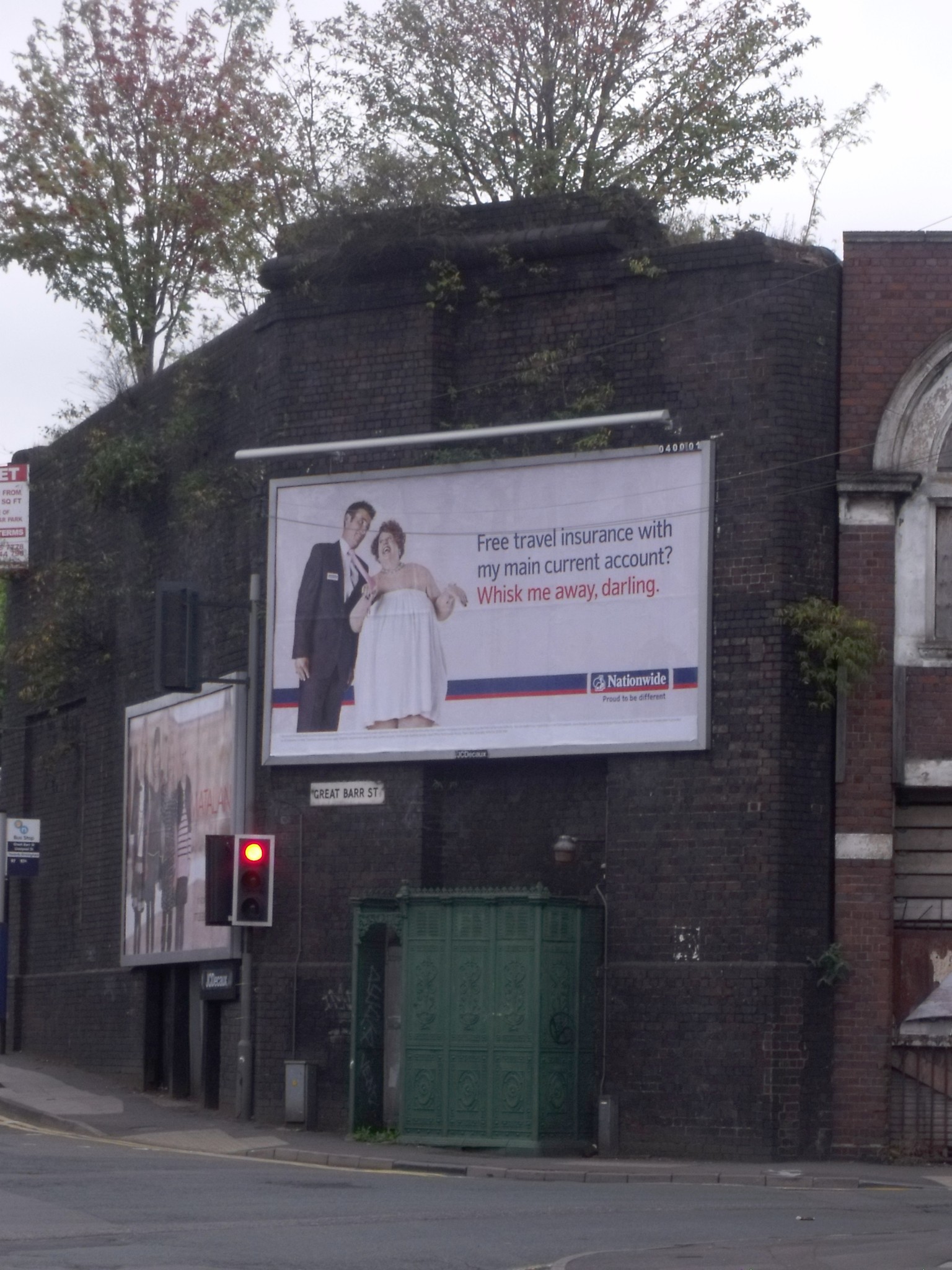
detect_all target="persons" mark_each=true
[130,718,192,955]
[290,500,377,733]
[350,519,468,732]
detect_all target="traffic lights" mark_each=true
[232,834,272,927]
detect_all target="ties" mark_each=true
[348,548,375,591]
